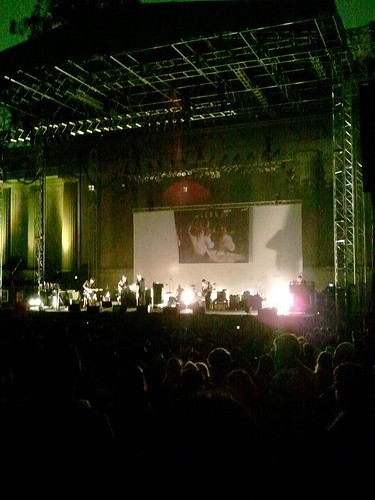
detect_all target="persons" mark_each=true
[1,277,375,500]
[187,221,236,260]
